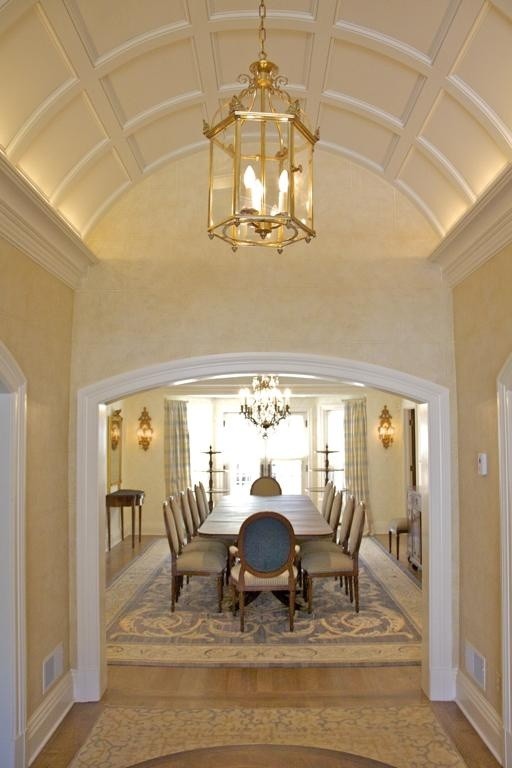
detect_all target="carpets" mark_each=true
[105,537,423,668]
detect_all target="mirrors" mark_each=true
[108,409,123,488]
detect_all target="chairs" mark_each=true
[387,515,408,560]
[229,512,299,632]
[163,477,364,613]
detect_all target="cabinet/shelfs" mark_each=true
[406,490,422,571]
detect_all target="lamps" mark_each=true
[237,375,291,439]
[111,420,121,450]
[377,405,395,449]
[137,407,154,451]
[199,1,323,252]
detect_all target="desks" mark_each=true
[106,490,145,549]
